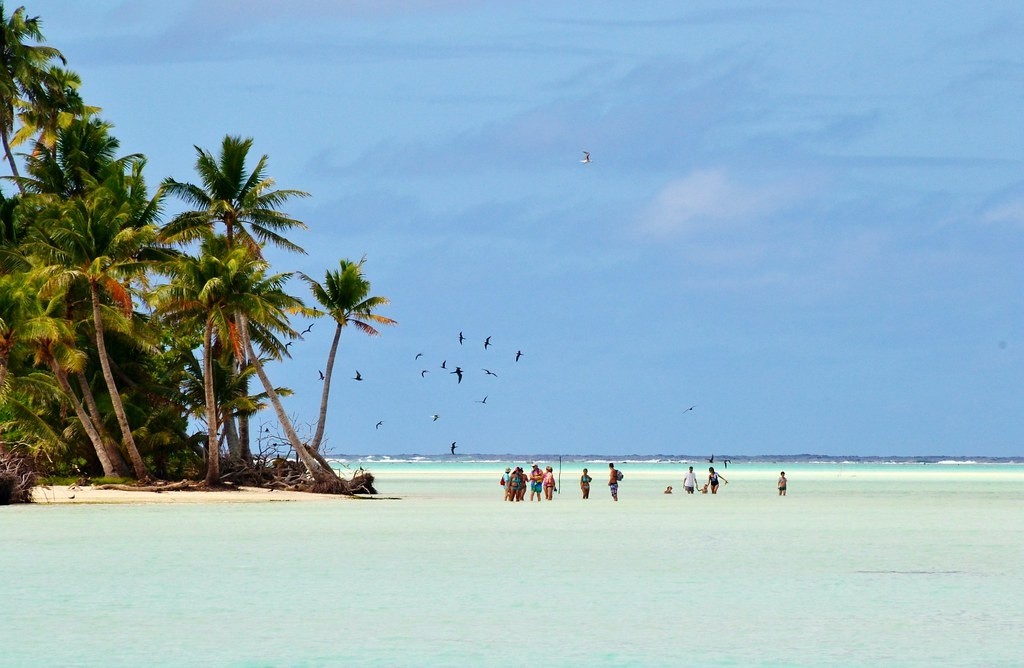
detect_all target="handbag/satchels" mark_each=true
[500,477,505,485]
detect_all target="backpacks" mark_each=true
[615,470,623,481]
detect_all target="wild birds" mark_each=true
[580,151,593,166]
[682,406,696,415]
[286,307,524,456]
[706,453,732,469]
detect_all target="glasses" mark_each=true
[689,469,693,470]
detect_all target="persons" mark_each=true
[517,468,532,501]
[580,469,592,499]
[608,462,619,501]
[697,483,708,493]
[663,486,673,495]
[509,467,521,501]
[683,467,698,494]
[500,467,510,500]
[530,463,544,501]
[543,466,556,499]
[544,467,556,500]
[778,472,787,496]
[708,467,728,494]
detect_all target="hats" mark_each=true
[504,467,511,473]
[609,463,614,467]
[531,462,538,467]
